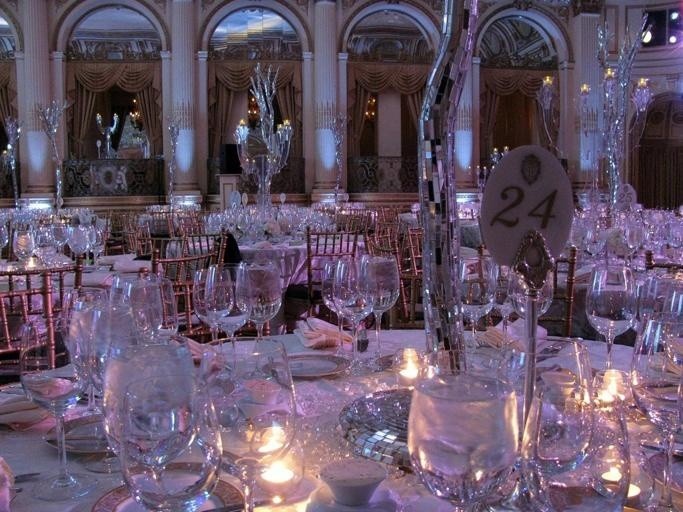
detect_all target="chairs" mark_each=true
[0,189,682,392]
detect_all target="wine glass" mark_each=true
[1,199,682,512]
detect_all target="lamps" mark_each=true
[535,6,661,205]
[231,58,295,219]
[0,96,180,209]
